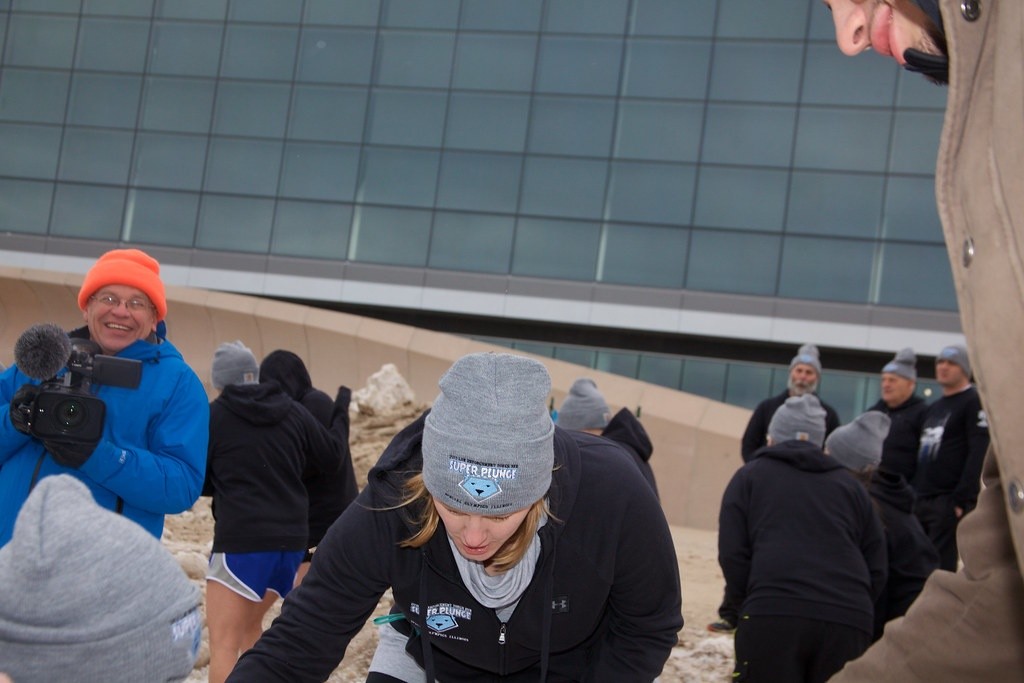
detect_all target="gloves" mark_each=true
[35,435,97,469]
[329,385,352,425]
[10,384,40,435]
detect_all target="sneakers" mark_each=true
[708,617,737,634]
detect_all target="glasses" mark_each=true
[90,295,155,318]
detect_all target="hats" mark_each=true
[556,378,611,429]
[422,353,554,516]
[765,393,827,448]
[936,346,971,377]
[882,347,916,384]
[211,339,259,387]
[824,411,893,474]
[78,249,168,323]
[0,474,202,683]
[789,344,820,377]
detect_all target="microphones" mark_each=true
[13,323,72,381]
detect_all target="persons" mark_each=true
[706,345,990,635]
[716,393,940,683]
[819,0,1024,683]
[0,249,210,683]
[556,377,653,484]
[224,352,684,683]
[198,341,352,683]
[258,348,358,589]
